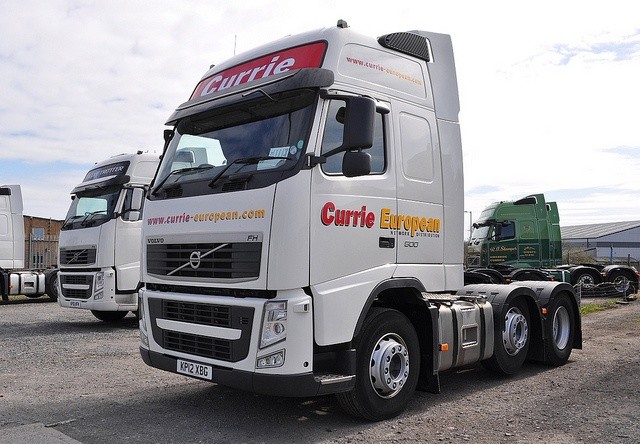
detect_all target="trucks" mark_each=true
[55,145,207,321]
[466,194,639,297]
[137,19,584,421]
[1,185,64,301]
